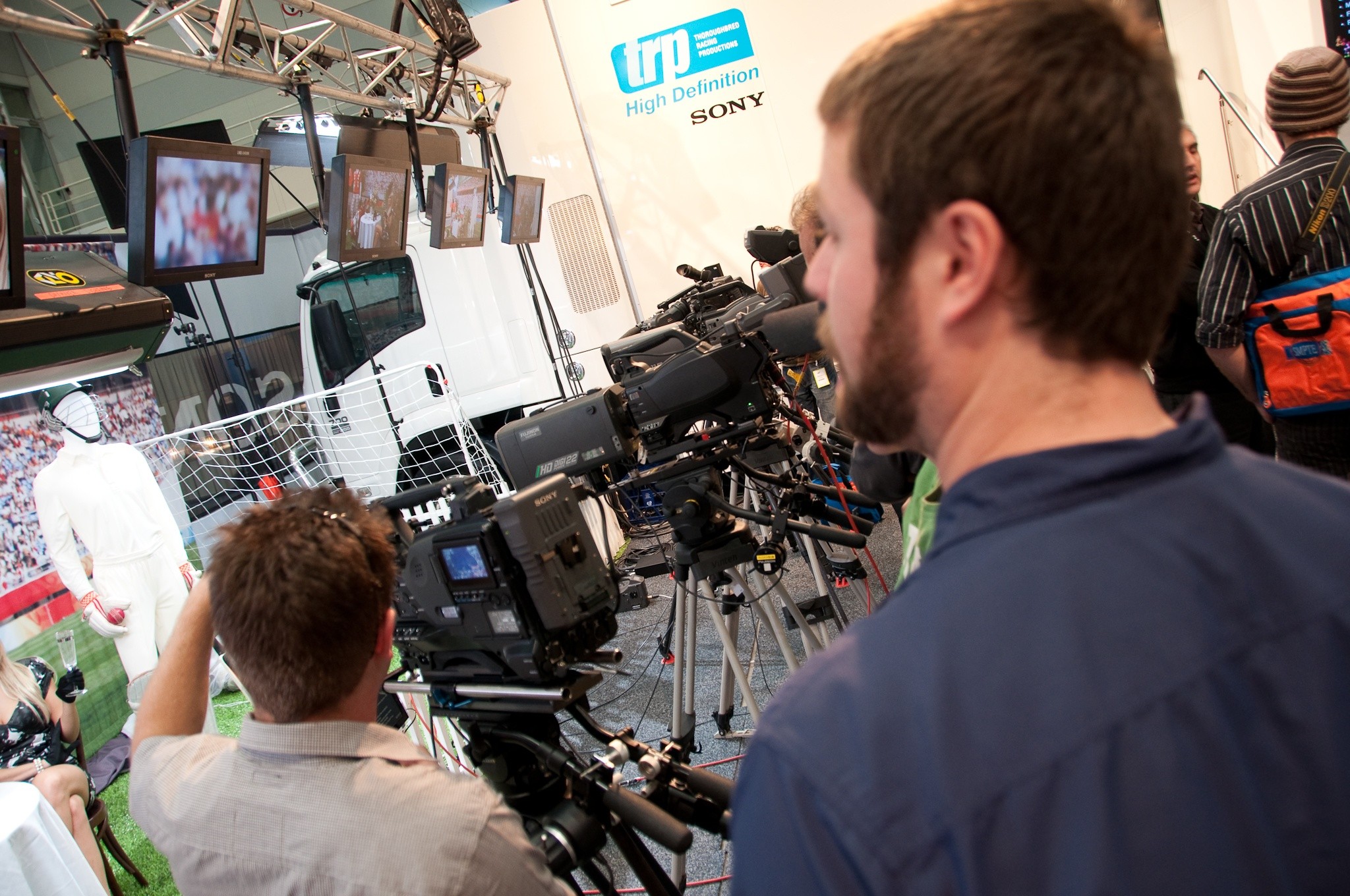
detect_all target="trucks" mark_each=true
[298,0,1330,537]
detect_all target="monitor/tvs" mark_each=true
[326,153,413,262]
[501,175,546,244]
[429,162,490,249]
[126,135,272,287]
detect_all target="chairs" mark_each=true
[16,656,149,896]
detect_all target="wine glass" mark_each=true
[54,629,90,698]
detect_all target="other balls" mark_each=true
[107,607,125,625]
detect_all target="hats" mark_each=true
[1266,47,1350,132]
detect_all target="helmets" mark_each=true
[38,382,92,415]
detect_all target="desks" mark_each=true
[0,781,109,896]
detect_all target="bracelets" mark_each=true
[34,757,43,773]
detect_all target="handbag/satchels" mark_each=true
[1238,267,1350,416]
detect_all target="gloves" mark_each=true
[56,667,84,704]
[180,563,200,589]
[45,719,80,767]
[80,591,131,639]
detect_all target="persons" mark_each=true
[0,635,111,896]
[450,198,456,233]
[466,186,480,240]
[370,195,397,247]
[792,189,941,591]
[703,0,1350,896]
[1197,45,1349,481]
[154,176,258,273]
[34,383,218,738]
[122,487,576,896]
[1156,126,1277,458]
